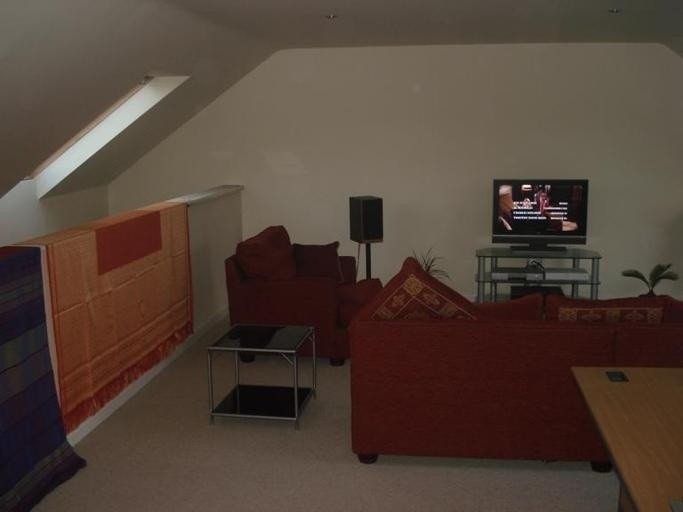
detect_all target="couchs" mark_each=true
[347,319,683,473]
[224,226,356,366]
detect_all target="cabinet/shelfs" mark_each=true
[475,247,603,304]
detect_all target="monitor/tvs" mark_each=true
[491,179,589,252]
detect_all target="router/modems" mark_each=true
[545,268,588,281]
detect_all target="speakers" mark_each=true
[350,195,384,242]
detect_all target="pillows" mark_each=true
[545,295,673,323]
[473,293,543,322]
[236,226,293,279]
[661,294,683,323]
[355,257,487,321]
[261,259,296,280]
[291,241,345,284]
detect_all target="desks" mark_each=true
[570,366,683,512]
